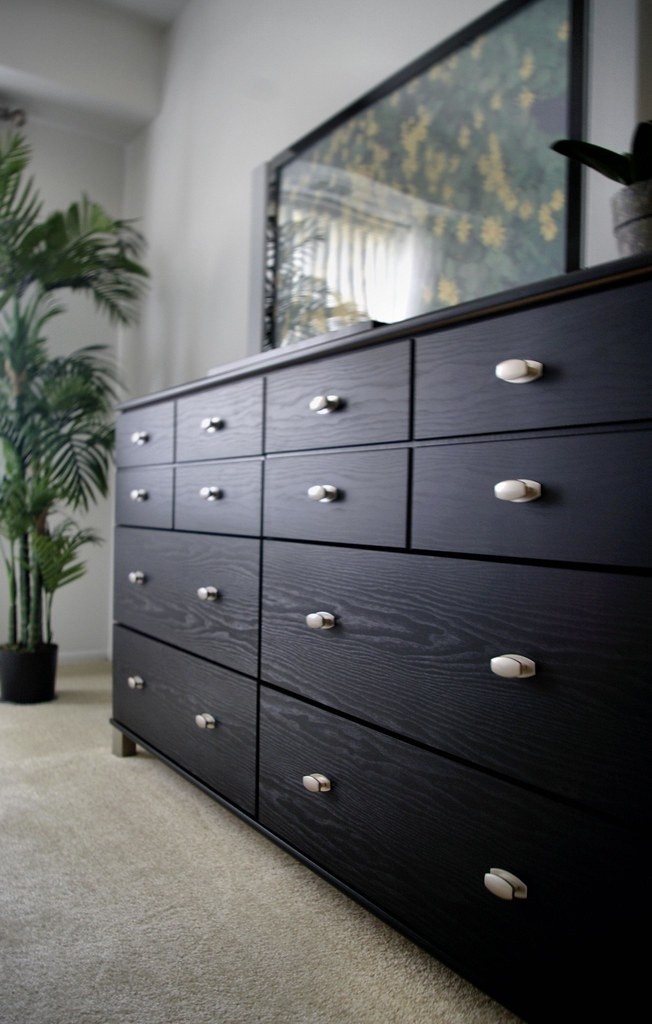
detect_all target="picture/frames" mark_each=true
[260,0,591,354]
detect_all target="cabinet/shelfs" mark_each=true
[110,250,652,1024]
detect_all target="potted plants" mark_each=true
[0,131,153,704]
